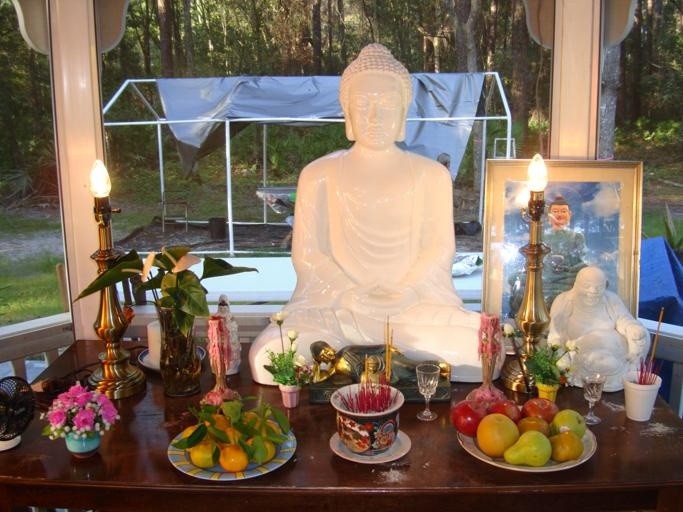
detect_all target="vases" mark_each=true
[279,383,301,408]
[65,429,100,453]
[155,296,201,398]
[536,382,559,402]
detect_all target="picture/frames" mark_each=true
[482,158,644,387]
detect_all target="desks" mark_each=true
[0,339,683,511]
[256,188,298,248]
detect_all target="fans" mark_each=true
[0,376,35,452]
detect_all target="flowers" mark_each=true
[39,384,121,441]
[263,312,314,388]
[72,246,259,339]
[502,324,580,385]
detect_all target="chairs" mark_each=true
[162,191,188,232]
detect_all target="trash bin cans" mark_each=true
[208,218,227,239]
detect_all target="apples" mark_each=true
[524,397,556,419]
[490,398,521,424]
[448,396,489,439]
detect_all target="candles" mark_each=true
[146,319,163,368]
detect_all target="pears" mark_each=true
[493,430,551,463]
[549,410,586,439]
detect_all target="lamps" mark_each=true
[499,153,549,393]
[82,159,147,399]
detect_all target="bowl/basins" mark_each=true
[330,383,405,456]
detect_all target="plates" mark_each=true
[456,406,598,474]
[138,346,206,370]
[330,430,411,464]
[167,425,296,481]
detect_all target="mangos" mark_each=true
[550,431,583,461]
[520,416,548,433]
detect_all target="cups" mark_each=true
[622,371,662,421]
[147,320,161,357]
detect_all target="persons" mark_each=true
[309,341,451,384]
[247,42,507,386]
[210,294,242,376]
[507,197,610,320]
[546,266,651,393]
[360,355,385,384]
[436,152,451,169]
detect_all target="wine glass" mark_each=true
[582,374,606,425]
[415,364,440,422]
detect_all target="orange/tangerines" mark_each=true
[184,414,280,470]
